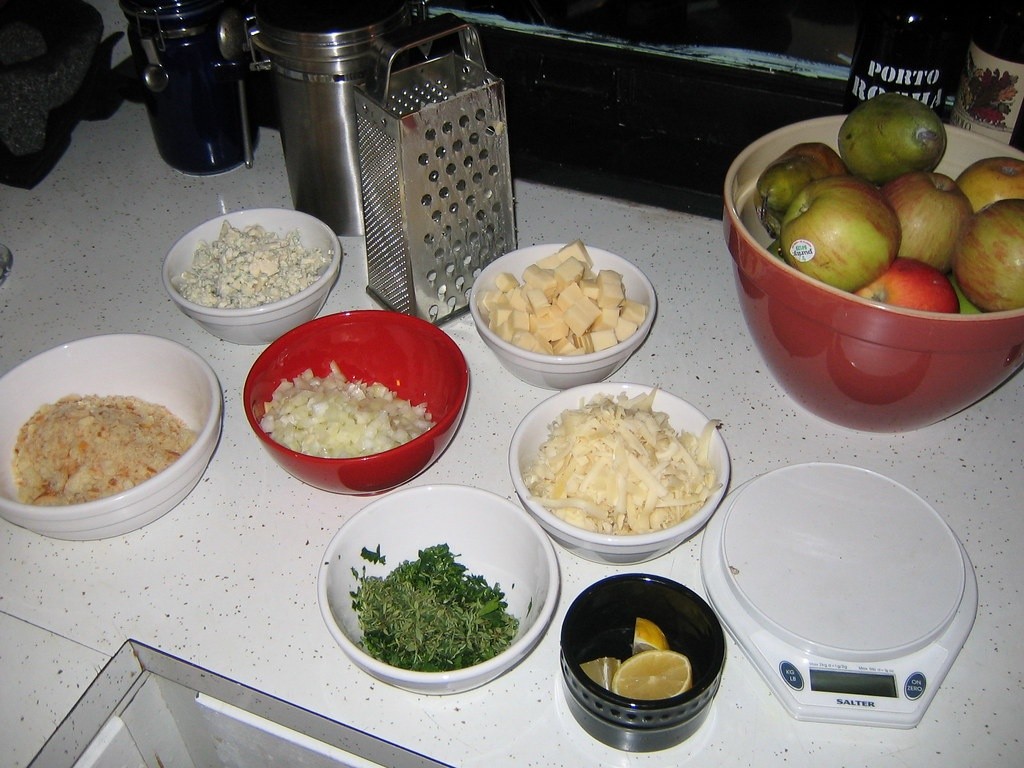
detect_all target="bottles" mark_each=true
[844,0,969,123]
[118,0,260,177]
[949,0,1024,153]
[243,0,426,237]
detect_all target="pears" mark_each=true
[756,153,830,222]
[838,92,946,189]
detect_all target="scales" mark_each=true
[699,461,981,728]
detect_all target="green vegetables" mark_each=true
[350,543,520,673]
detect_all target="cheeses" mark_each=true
[483,239,648,357]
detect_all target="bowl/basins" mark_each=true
[561,573,727,753]
[162,207,343,345]
[0,334,223,541]
[316,484,561,695]
[242,310,469,497]
[725,114,1024,435]
[508,382,731,566]
[469,242,659,390]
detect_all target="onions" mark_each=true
[259,360,437,458]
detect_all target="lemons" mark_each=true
[575,617,690,700]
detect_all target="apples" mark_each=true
[753,142,1024,313]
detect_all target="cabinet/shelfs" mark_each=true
[74,675,378,768]
